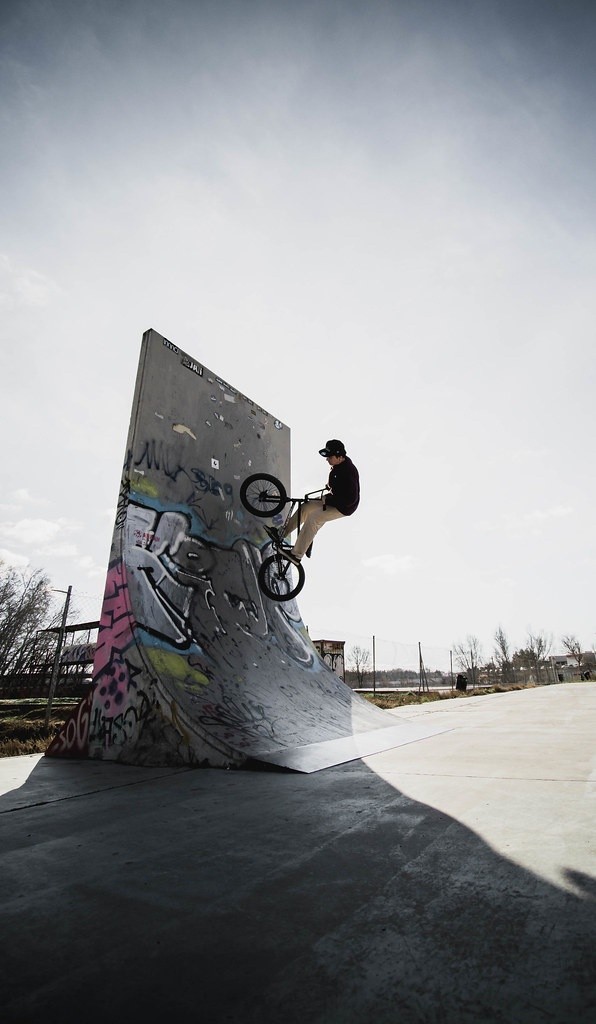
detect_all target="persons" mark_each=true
[262,439,360,566]
[581,668,593,681]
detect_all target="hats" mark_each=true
[318,439,346,457]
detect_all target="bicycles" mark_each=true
[239,472,329,602]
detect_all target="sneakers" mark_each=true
[263,525,283,544]
[277,547,301,566]
